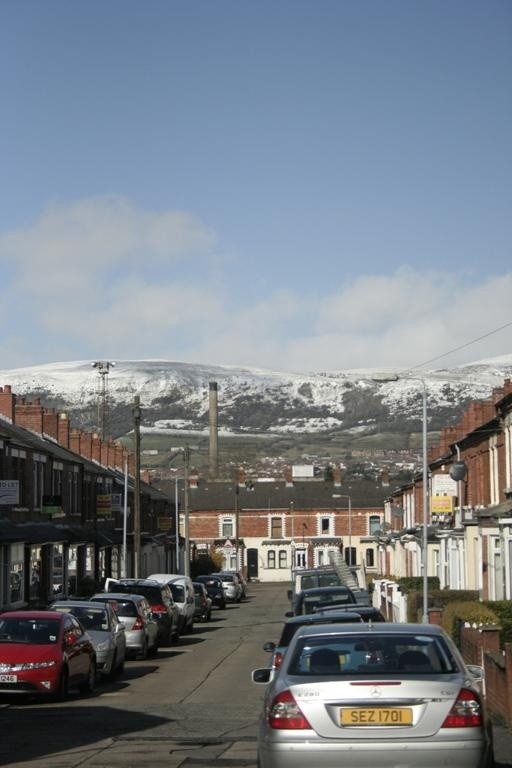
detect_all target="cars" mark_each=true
[192,568,248,623]
[46,600,127,684]
[0,610,99,704]
[262,560,387,671]
[248,620,491,767]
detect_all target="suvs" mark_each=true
[88,592,163,662]
[107,577,181,652]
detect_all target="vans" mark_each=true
[146,573,195,638]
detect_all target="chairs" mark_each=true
[8,623,34,640]
[309,647,434,673]
[93,612,106,624]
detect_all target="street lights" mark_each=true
[369,370,432,627]
[91,360,117,443]
[331,492,353,567]
